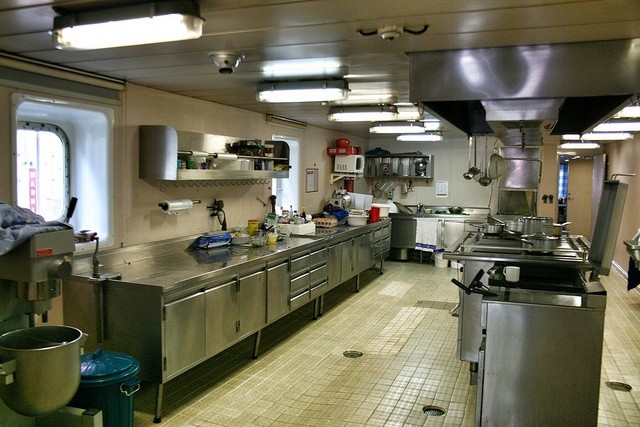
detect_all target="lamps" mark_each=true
[395,132,443,142]
[367,120,427,134]
[326,105,397,124]
[265,114,309,133]
[253,78,350,104]
[47,1,207,54]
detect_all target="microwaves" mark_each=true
[334,154,365,173]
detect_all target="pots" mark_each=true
[552,222,572,237]
[225,139,262,152]
[513,232,560,254]
[485,223,516,235]
[486,213,523,233]
[523,216,554,235]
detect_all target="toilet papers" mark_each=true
[161,198,192,214]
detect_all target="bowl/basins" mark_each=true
[322,211,345,224]
[448,207,463,214]
[277,233,287,241]
[347,214,370,226]
[335,138,350,148]
[252,228,268,247]
[424,209,432,214]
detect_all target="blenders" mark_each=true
[1,228,104,427]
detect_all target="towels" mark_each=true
[413,218,438,262]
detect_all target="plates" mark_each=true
[230,233,250,244]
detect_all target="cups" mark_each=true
[503,265,520,282]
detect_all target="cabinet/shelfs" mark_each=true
[441,219,466,252]
[62,267,239,423]
[390,220,419,249]
[329,171,365,185]
[239,250,289,359]
[288,240,329,321]
[364,154,435,180]
[374,221,392,273]
[356,225,374,290]
[327,232,355,292]
[138,123,291,181]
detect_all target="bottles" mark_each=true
[263,145,275,157]
[255,159,265,170]
[282,210,289,223]
[265,160,275,169]
[289,204,308,223]
[177,158,214,169]
[248,220,258,236]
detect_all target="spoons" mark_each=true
[479,136,492,186]
[462,136,474,180]
[469,136,480,175]
